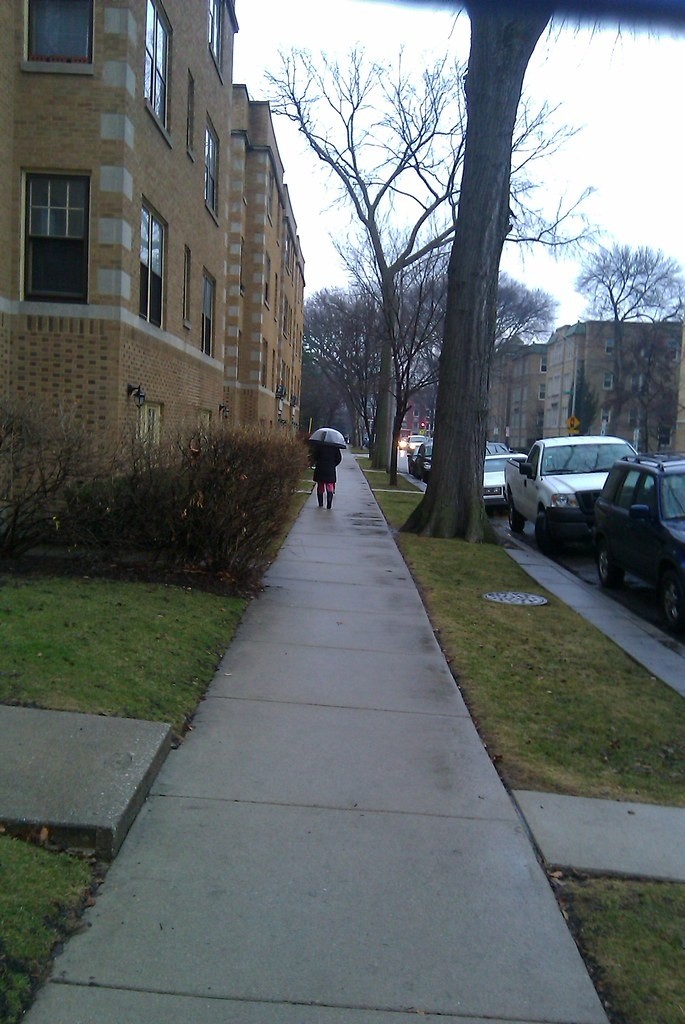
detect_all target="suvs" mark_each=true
[593,451,685,639]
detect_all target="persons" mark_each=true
[309,441,342,509]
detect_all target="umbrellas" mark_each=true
[309,427,348,449]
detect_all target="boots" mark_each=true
[318,493,323,506]
[327,493,333,509]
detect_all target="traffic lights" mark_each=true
[419,415,426,429]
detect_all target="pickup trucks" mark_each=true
[502,434,643,559]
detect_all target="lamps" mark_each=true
[219,404,230,419]
[128,382,145,409]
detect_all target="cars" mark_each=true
[482,453,528,507]
[399,434,512,483]
